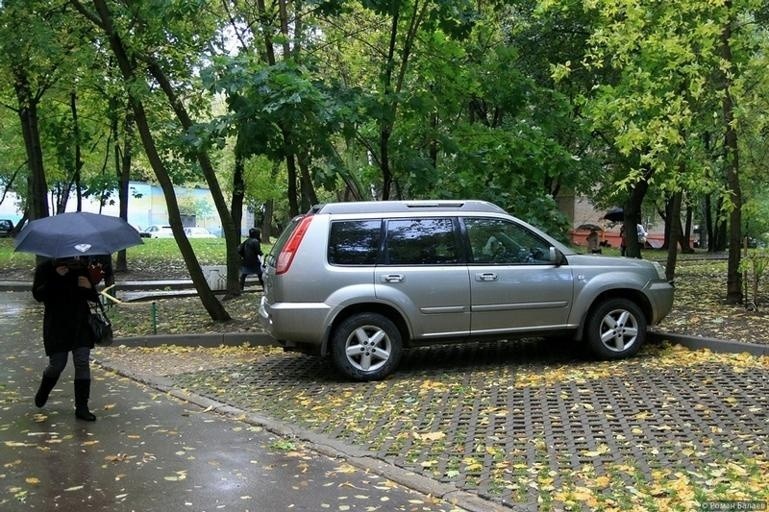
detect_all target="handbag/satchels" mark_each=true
[238,244,244,254]
[92,314,112,347]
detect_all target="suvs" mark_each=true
[260,199,675,377]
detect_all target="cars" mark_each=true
[142,225,215,240]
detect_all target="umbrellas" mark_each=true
[577,223,603,233]
[602,207,624,222]
[14,210,146,276]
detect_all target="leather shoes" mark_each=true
[35,393,48,407]
[76,408,96,421]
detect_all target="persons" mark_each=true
[32,256,99,420]
[240,227,263,290]
[620,225,626,257]
[586,229,602,254]
[636,222,646,259]
